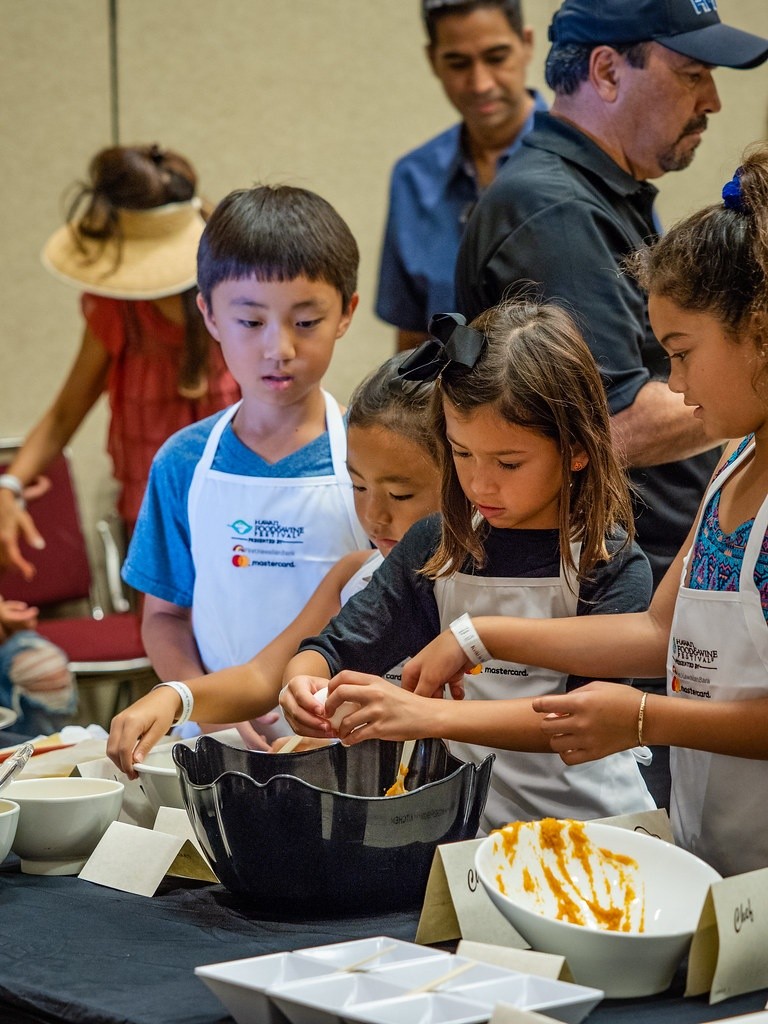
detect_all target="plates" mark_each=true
[0,705,18,730]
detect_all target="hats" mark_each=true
[40,178,209,300]
[547,0,768,71]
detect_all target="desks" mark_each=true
[0,730,768,1024]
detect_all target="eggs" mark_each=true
[313,687,361,731]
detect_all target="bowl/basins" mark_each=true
[172,734,496,920]
[473,820,725,999]
[131,750,186,815]
[0,777,125,876]
[0,798,21,866]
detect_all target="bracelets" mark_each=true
[450,613,492,665]
[152,681,194,727]
[279,682,288,718]
[0,474,24,497]
[638,692,649,746]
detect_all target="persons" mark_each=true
[0,596,79,736]
[456,0,768,587]
[0,144,243,581]
[375,0,547,353]
[281,299,654,832]
[109,343,441,778]
[119,186,373,745]
[401,153,767,876]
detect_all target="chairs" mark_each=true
[1,437,158,729]
